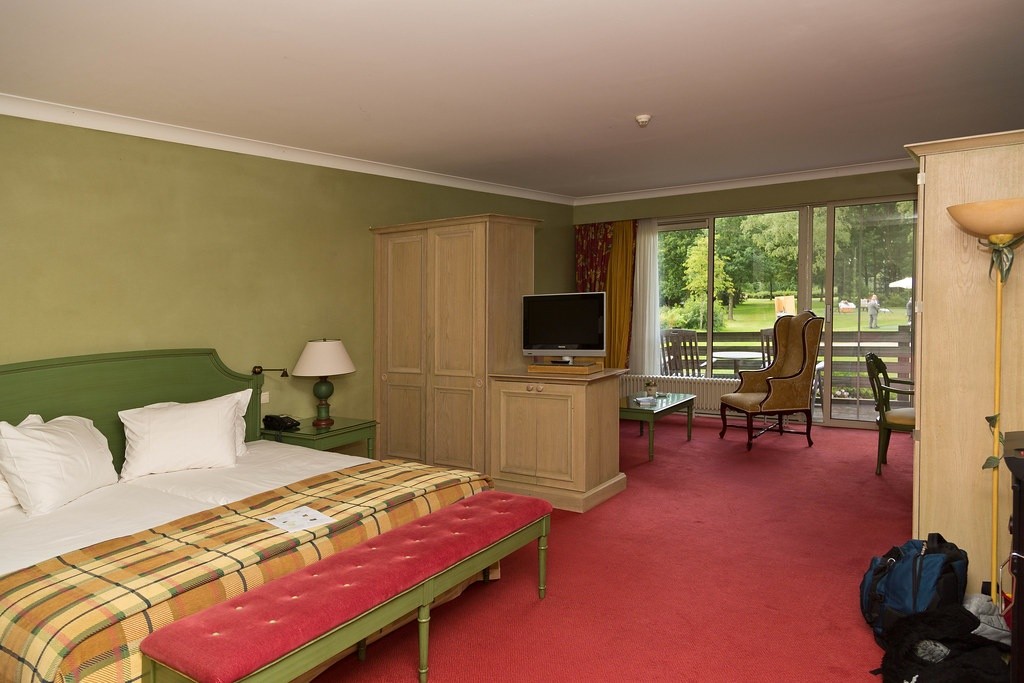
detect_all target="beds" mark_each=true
[0,349,494,683]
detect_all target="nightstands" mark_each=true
[261,415,376,461]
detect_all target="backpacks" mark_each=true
[859,533,1012,683]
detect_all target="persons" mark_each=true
[868,297,879,328]
[907,296,912,324]
[872,295,879,306]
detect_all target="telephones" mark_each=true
[262,413,302,430]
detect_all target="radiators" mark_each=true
[619,375,777,418]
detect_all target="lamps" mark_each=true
[293,337,357,427]
[947,199,1024,606]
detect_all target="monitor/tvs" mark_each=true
[522,293,608,367]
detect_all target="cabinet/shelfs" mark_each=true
[485,369,632,513]
[905,127,1024,595]
[370,213,544,496]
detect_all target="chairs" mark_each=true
[758,327,827,419]
[718,311,819,449]
[865,350,924,476]
[661,330,717,378]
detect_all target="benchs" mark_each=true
[139,493,553,683]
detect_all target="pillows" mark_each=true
[110,386,254,485]
[0,416,118,514]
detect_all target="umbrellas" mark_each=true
[889,277,912,289]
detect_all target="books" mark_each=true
[635,396,657,406]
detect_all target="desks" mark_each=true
[620,391,697,461]
[711,351,768,379]
